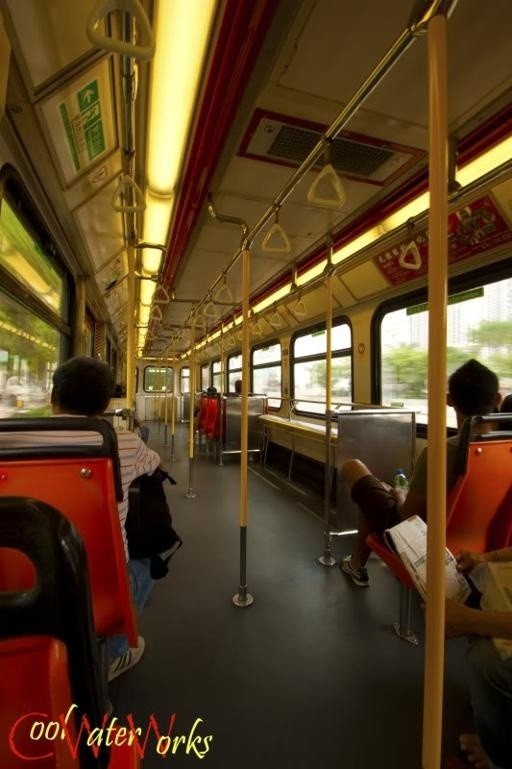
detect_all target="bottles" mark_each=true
[393,468,410,501]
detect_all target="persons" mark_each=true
[235,380,242,394]
[111,384,150,443]
[191,386,218,439]
[1,356,161,683]
[335,359,501,589]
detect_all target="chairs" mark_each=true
[199,398,213,457]
[0,417,138,717]
[209,398,219,459]
[366,412,512,647]
[0,495,141,769]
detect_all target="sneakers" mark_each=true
[108,636,144,680]
[341,554,370,587]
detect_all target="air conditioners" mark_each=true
[244,114,415,185]
